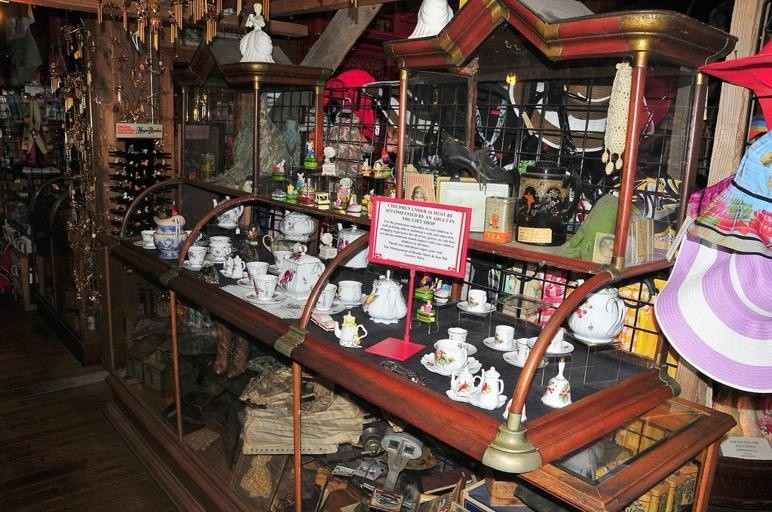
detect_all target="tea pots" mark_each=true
[224,255,245,279]
[279,209,318,243]
[336,222,371,268]
[210,194,246,231]
[152,214,189,259]
[237,250,367,317]
[334,311,368,348]
[567,278,625,344]
[361,268,410,326]
[450,366,483,398]
[510,162,581,245]
[474,366,505,410]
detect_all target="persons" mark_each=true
[409,185,429,204]
[237,2,276,63]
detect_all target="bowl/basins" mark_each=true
[434,293,450,304]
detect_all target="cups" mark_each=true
[261,234,309,257]
[140,228,231,265]
[541,324,565,350]
[447,327,468,342]
[494,324,514,348]
[468,289,488,310]
[515,338,531,363]
[434,339,468,371]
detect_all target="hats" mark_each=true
[698,41,772,132]
[654,225,772,395]
[687,130,772,259]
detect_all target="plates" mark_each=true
[181,260,214,271]
[456,301,496,315]
[420,352,483,376]
[483,336,518,352]
[503,353,551,370]
[526,337,575,355]
[434,339,477,356]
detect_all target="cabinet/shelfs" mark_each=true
[98,1,736,510]
